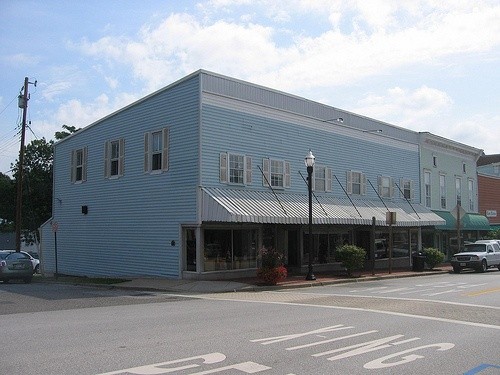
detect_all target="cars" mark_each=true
[392,243,424,256]
[0,249,40,283]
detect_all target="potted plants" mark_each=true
[257,248,288,286]
[412,251,427,272]
[336,244,366,277]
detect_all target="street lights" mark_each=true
[304,149,317,280]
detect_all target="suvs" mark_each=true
[374,239,390,259]
[450,240,500,272]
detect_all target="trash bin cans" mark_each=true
[411,255,427,271]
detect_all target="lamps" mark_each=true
[323,118,343,122]
[363,129,382,133]
